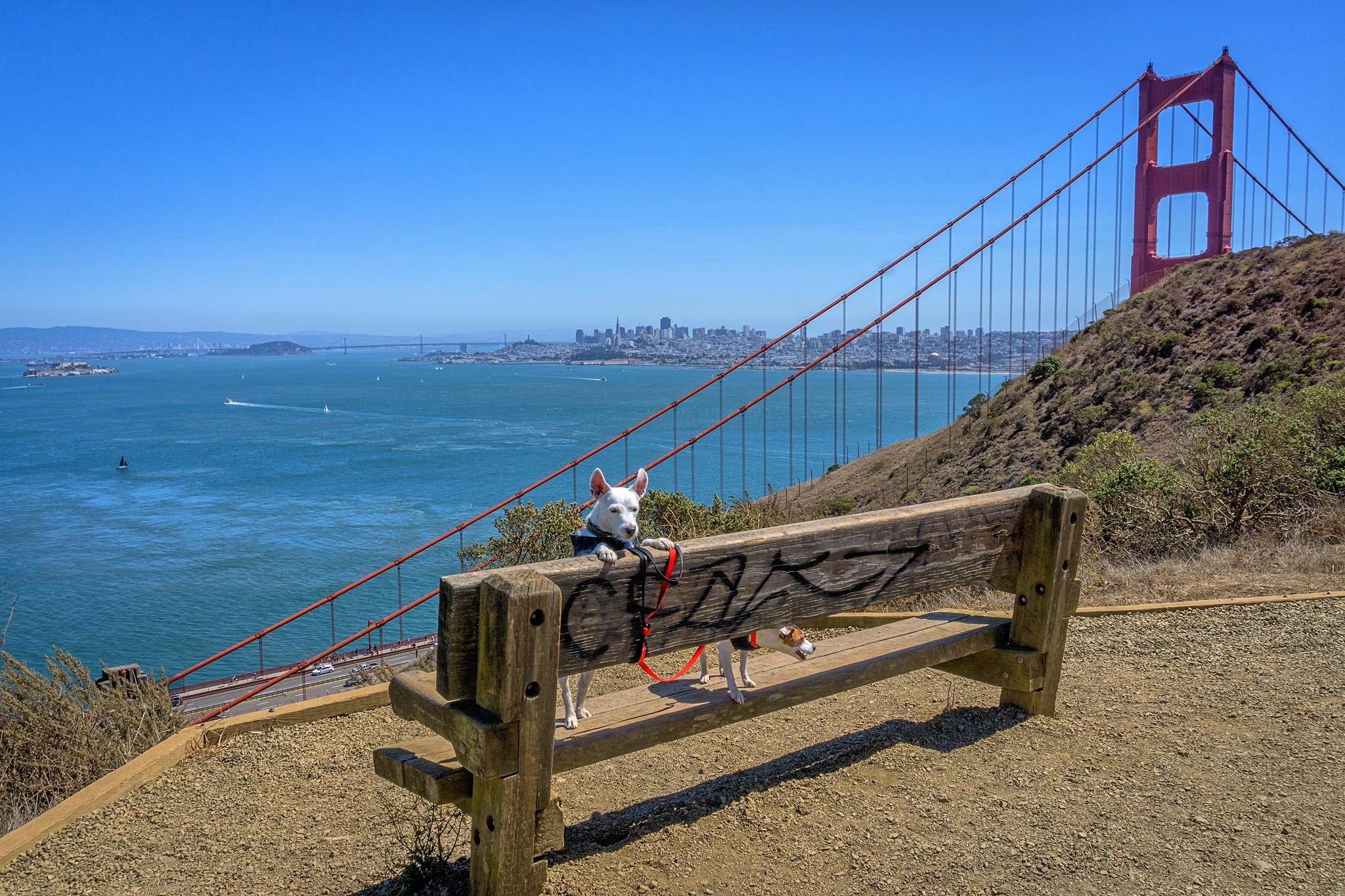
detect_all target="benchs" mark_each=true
[373,480,1089,896]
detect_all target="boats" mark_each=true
[27,382,43,387]
[438,366,441,370]
[332,363,335,365]
[600,377,606,380]
[225,398,232,403]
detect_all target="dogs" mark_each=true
[558,467,675,729]
[698,625,816,704]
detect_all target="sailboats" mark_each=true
[242,375,244,379]
[323,403,330,413]
[115,454,131,469]
[377,376,380,380]
